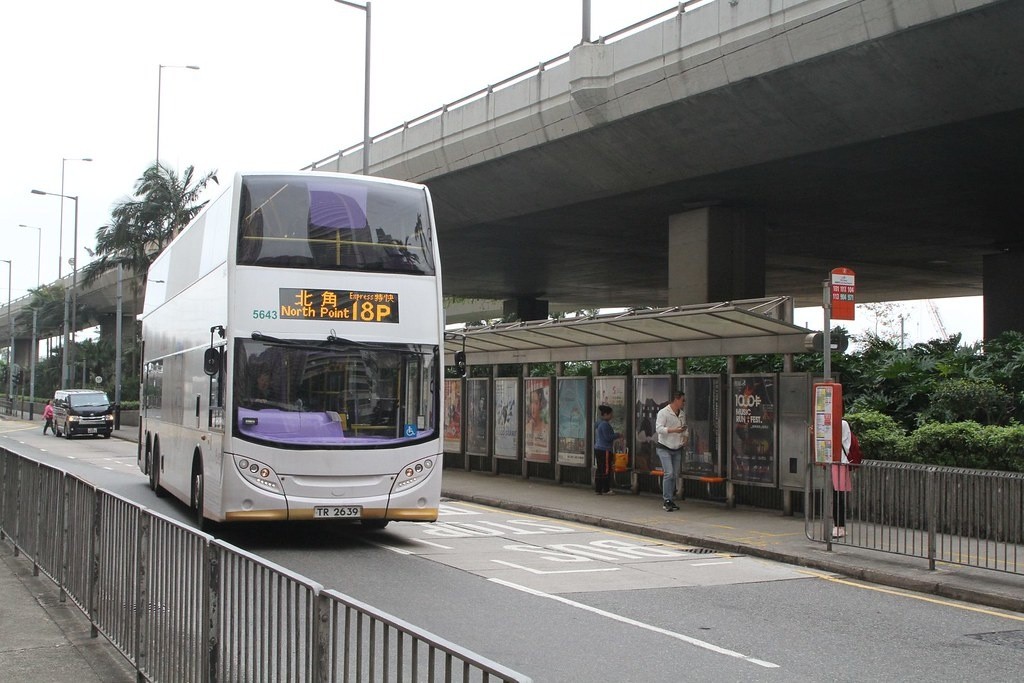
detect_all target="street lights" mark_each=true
[30,190,78,389]
[156,65,201,176]
[18,225,42,421]
[58,157,93,280]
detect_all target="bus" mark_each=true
[137,170,467,536]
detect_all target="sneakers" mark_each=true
[662,499,673,512]
[671,502,680,510]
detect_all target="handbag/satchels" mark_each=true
[615,448,629,472]
[48,420,53,427]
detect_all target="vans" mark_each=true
[52,388,116,439]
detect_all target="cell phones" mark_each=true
[681,425,689,430]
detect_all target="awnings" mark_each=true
[442,296,814,354]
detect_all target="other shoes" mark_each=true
[832,527,847,537]
[603,490,615,495]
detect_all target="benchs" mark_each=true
[651,470,728,501]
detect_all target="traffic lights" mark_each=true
[16,370,24,384]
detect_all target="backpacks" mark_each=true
[842,430,862,469]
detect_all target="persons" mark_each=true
[373,383,394,435]
[656,391,689,512]
[808,398,850,538]
[525,388,549,434]
[249,371,278,401]
[593,406,623,494]
[41,400,56,435]
